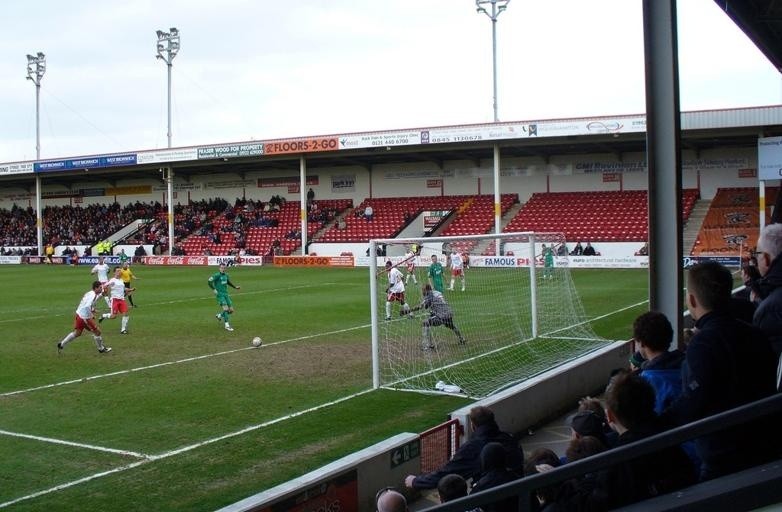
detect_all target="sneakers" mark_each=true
[224,326,234,331]
[121,330,127,334]
[385,316,392,320]
[458,337,466,344]
[98,347,112,353]
[99,314,104,323]
[422,344,434,351]
[216,314,221,323]
[446,286,466,292]
[58,343,63,355]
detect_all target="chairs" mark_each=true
[0,188,701,256]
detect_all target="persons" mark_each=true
[1,185,286,266]
[287,228,301,239]
[92,255,112,311]
[386,261,414,319]
[207,263,241,332]
[57,282,113,354]
[120,261,141,308]
[428,255,449,292]
[403,249,419,284]
[377,224,781,509]
[307,188,349,230]
[99,267,136,335]
[399,284,466,348]
[447,247,466,292]
[364,202,376,222]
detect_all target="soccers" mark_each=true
[253,338,262,348]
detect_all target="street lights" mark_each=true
[24,51,47,259]
[154,26,183,256]
[475,0,512,256]
